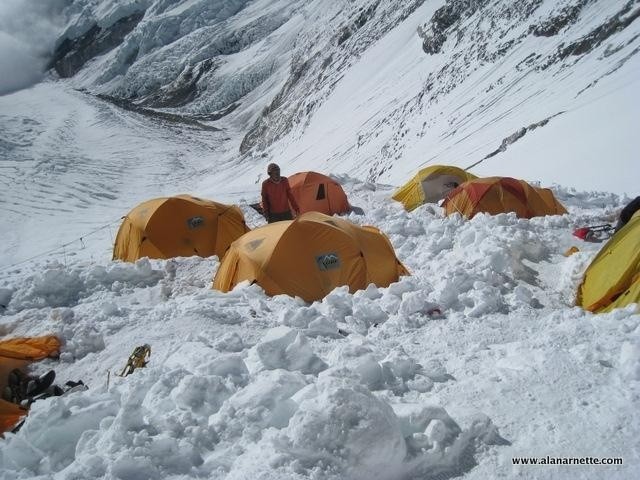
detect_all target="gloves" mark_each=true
[295,208,300,217]
[266,215,272,221]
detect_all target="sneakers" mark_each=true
[27,371,55,397]
[21,393,47,409]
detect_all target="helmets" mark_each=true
[268,163,280,172]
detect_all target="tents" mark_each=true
[111,193,251,264]
[0,336,59,438]
[287,171,352,221]
[439,176,569,221]
[390,164,479,212]
[574,196,640,315]
[211,211,411,304]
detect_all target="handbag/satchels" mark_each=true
[9,369,33,401]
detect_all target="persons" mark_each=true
[260,163,301,224]
